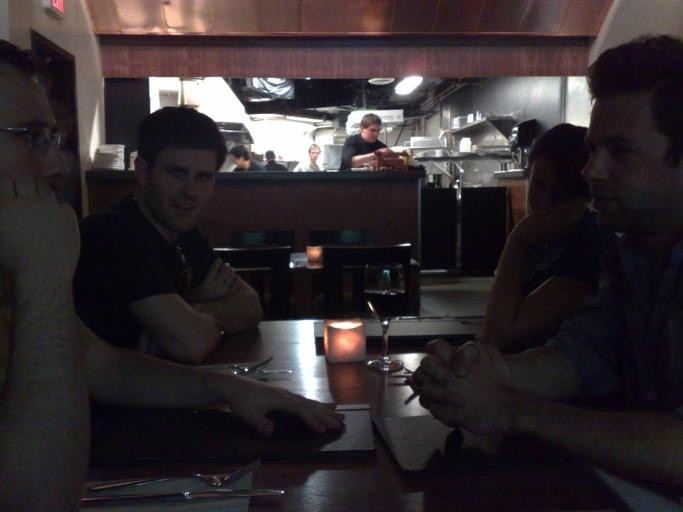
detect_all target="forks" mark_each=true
[89,461,262,490]
[193,355,273,374]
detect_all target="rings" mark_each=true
[224,280,232,288]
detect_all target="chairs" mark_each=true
[228,224,412,328]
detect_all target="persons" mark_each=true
[228,145,268,171]
[262,151,289,172]
[68,102,263,439]
[337,114,395,172]
[0,37,345,512]
[411,30,683,488]
[476,124,618,356]
[291,144,324,172]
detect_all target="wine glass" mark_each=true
[365,262,407,372]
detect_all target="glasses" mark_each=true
[2,123,61,145]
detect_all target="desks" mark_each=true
[87,321,637,511]
[222,259,422,317]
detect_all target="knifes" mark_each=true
[209,369,293,374]
[80,487,284,506]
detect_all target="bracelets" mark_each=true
[491,269,520,281]
[374,151,381,159]
